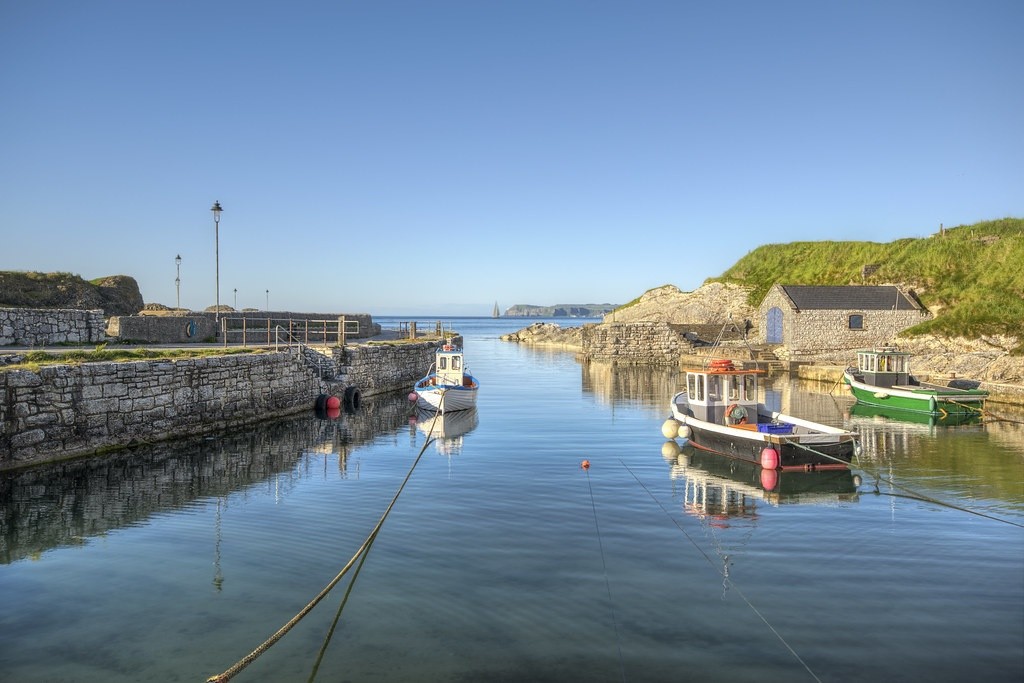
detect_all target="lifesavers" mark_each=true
[315,394,330,409]
[315,409,329,419]
[344,402,362,414]
[345,387,362,401]
[710,360,735,372]
[725,403,748,425]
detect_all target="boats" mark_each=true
[665,438,862,603]
[413,337,480,414]
[843,284,990,416]
[669,311,863,473]
[413,405,480,477]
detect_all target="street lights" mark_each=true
[233,288,238,311]
[175,254,182,310]
[209,199,225,336]
[266,290,269,311]
